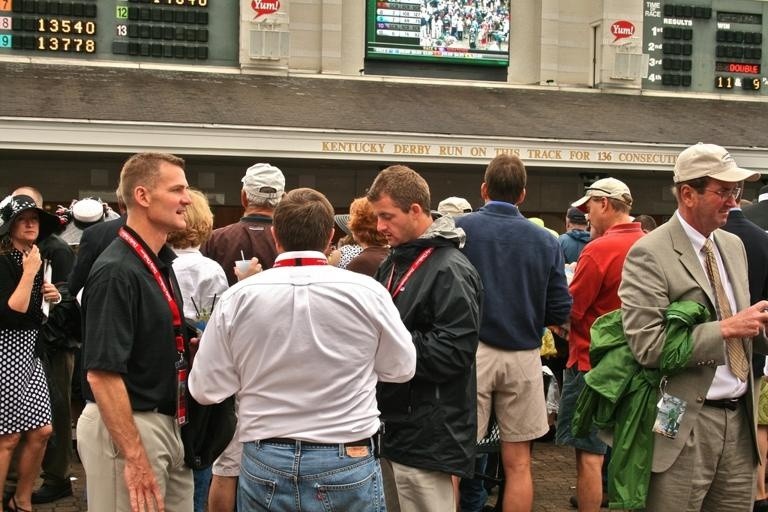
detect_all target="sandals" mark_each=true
[7,495,35,512]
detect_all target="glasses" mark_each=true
[705,187,742,200]
[584,186,612,195]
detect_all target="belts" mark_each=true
[140,402,179,417]
[257,430,380,449]
[703,397,741,411]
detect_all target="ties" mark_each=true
[701,238,749,384]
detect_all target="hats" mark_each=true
[238,162,286,201]
[566,206,586,220]
[333,214,355,240]
[0,194,62,248]
[58,198,121,246]
[570,176,633,214]
[672,140,762,184]
[526,215,561,241]
[428,195,473,218]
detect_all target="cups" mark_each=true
[233,259,251,280]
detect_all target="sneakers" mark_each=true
[569,490,609,508]
[30,479,73,505]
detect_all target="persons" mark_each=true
[419,0,510,51]
